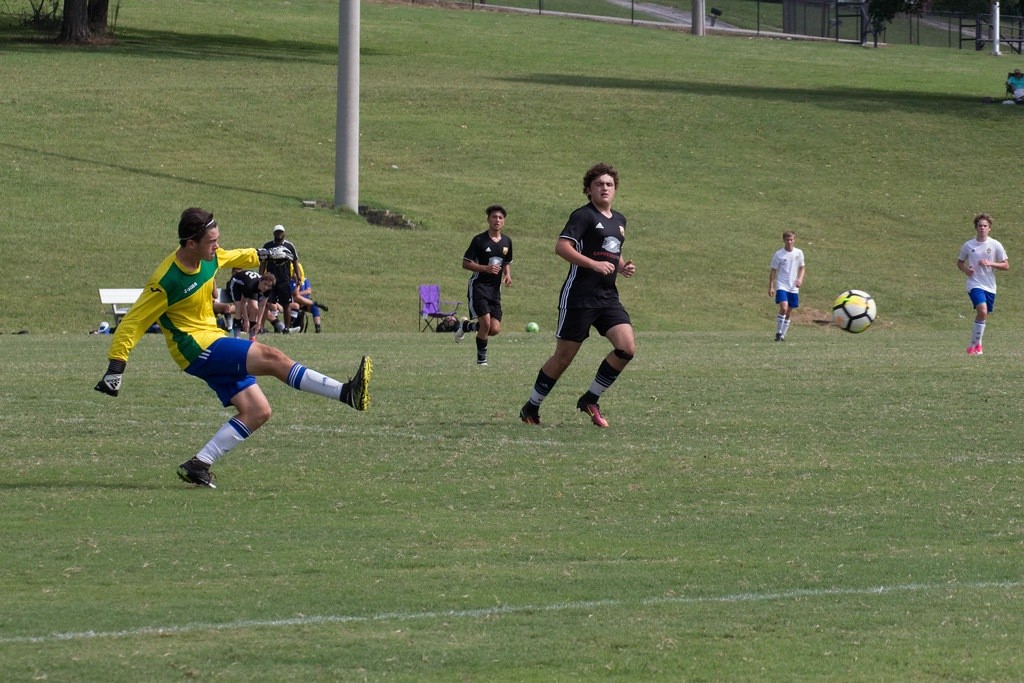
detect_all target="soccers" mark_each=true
[831,289,878,334]
[526,321,539,333]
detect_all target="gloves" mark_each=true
[257,246,294,262]
[94,359,126,398]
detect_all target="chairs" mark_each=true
[1006,72,1024,101]
[215,287,243,330]
[417,284,463,333]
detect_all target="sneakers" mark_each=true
[575,396,608,428]
[519,403,541,425]
[176,456,217,489]
[476,362,489,366]
[348,355,373,410]
[455,316,468,343]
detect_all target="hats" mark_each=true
[273,224,285,233]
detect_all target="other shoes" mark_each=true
[315,326,320,333]
[975,344,983,355]
[781,337,785,342]
[250,337,256,341]
[319,303,329,312]
[774,333,781,342]
[967,348,975,355]
[282,327,300,335]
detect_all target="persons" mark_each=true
[768,231,806,342]
[455,206,513,366]
[1005,68,1024,102]
[94,206,374,487]
[212,225,329,342]
[957,214,1010,355]
[520,163,636,427]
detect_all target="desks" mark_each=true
[98,288,146,330]
